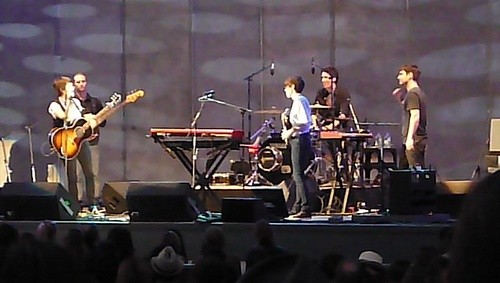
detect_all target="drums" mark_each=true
[249,135,318,188]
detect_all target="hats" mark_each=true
[356,251,382,263]
[38,222,56,235]
[150,247,184,276]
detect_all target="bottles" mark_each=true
[384,133,391,148]
[374,133,382,147]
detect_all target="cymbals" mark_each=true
[253,110,284,115]
[309,104,337,109]
[332,113,354,120]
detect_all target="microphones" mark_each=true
[199,89,215,100]
[311,57,316,74]
[270,63,274,75]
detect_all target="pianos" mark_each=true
[149,128,245,218]
[317,128,373,216]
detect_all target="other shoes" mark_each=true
[289,212,296,215]
[90,207,105,212]
[294,212,311,218]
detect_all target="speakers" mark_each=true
[0,182,81,221]
[126,182,206,222]
[102,182,130,214]
[380,168,435,214]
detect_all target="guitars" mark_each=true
[48,88,145,160]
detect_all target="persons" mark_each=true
[0,219,450,283]
[281,75,313,222]
[310,67,350,163]
[47,76,95,212]
[71,73,106,201]
[391,64,427,177]
